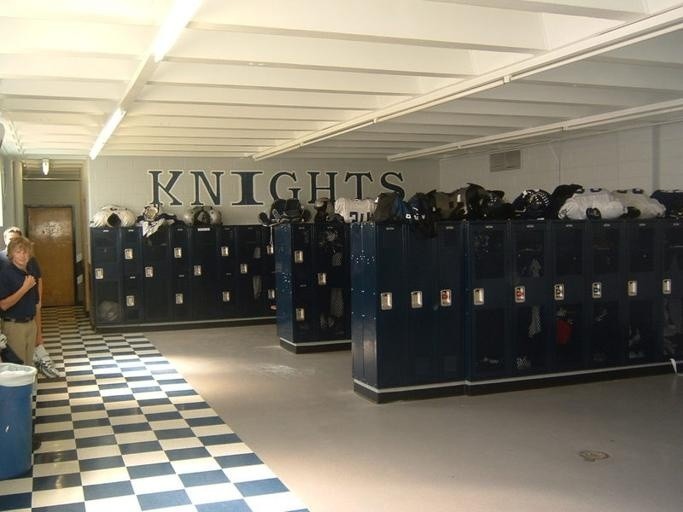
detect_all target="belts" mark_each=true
[1,315,35,323]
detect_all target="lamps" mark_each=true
[252,4,683,164]
[42,158,50,176]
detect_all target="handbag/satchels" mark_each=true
[96,301,124,324]
[88,203,224,238]
[256,184,683,227]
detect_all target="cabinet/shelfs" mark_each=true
[90,219,276,336]
[271,222,353,356]
[351,219,683,405]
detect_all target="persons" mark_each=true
[0,227,44,367]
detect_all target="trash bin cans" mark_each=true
[0,362,38,480]
